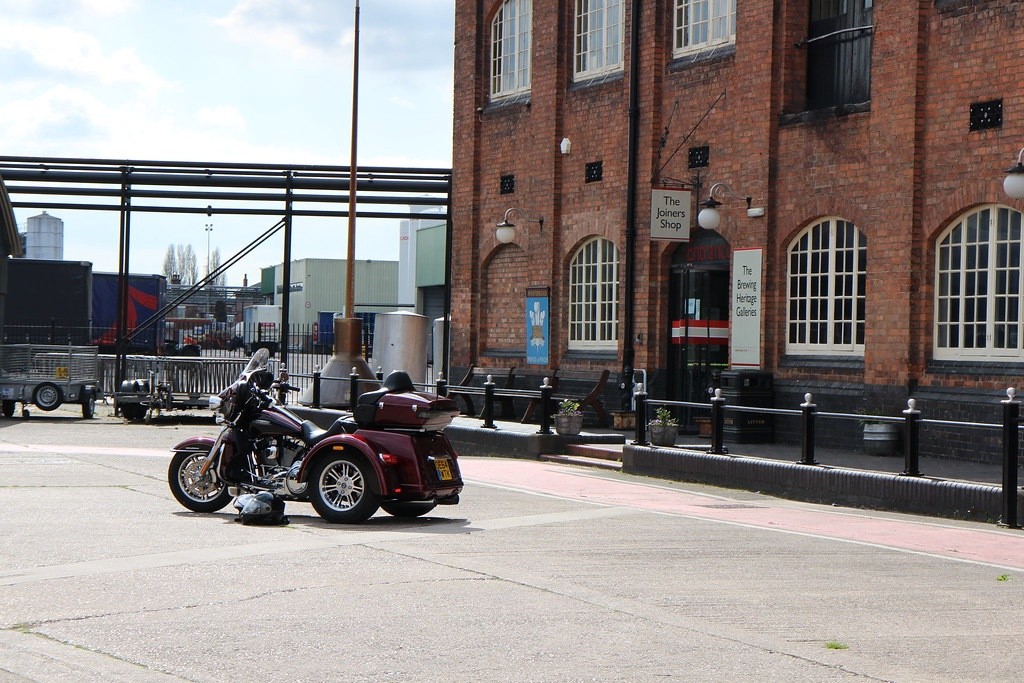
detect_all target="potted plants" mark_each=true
[853,402,898,456]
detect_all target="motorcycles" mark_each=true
[167,347,464,523]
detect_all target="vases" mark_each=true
[554,414,583,434]
[649,424,678,446]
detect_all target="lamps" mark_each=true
[1003,147,1024,199]
[698,183,751,230]
[495,208,543,244]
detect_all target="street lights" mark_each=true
[205,223,213,284]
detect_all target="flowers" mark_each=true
[647,407,678,427]
[550,399,583,418]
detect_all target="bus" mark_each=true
[164,318,213,348]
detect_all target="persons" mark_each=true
[184,334,201,356]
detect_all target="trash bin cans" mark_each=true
[721,370,775,443]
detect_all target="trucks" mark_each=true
[3,255,168,355]
[230,304,283,357]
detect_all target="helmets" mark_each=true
[233,491,290,525]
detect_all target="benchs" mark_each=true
[447,364,610,427]
